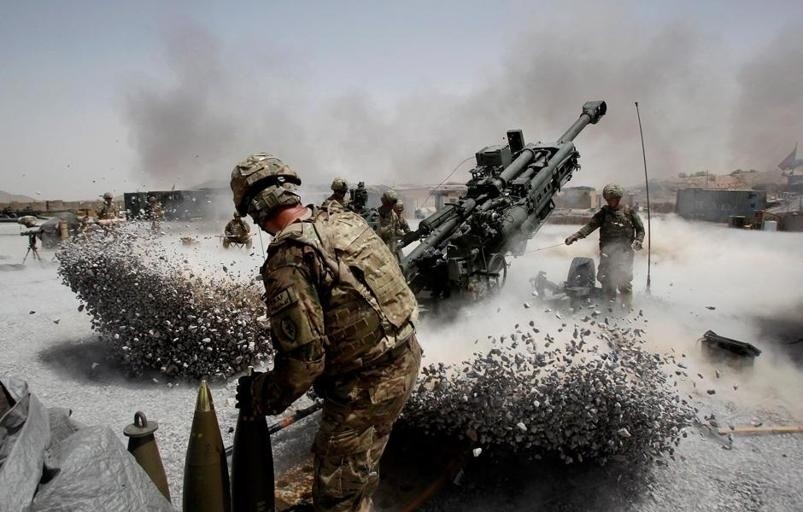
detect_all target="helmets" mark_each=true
[102,192,112,200]
[379,190,405,212]
[330,177,349,191]
[229,150,302,218]
[601,183,623,200]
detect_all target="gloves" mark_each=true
[233,370,267,412]
[632,239,643,251]
[564,235,578,245]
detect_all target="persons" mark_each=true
[393,199,409,235]
[93,192,120,240]
[325,176,348,214]
[372,187,403,259]
[563,181,646,317]
[228,149,426,510]
[220,211,252,251]
